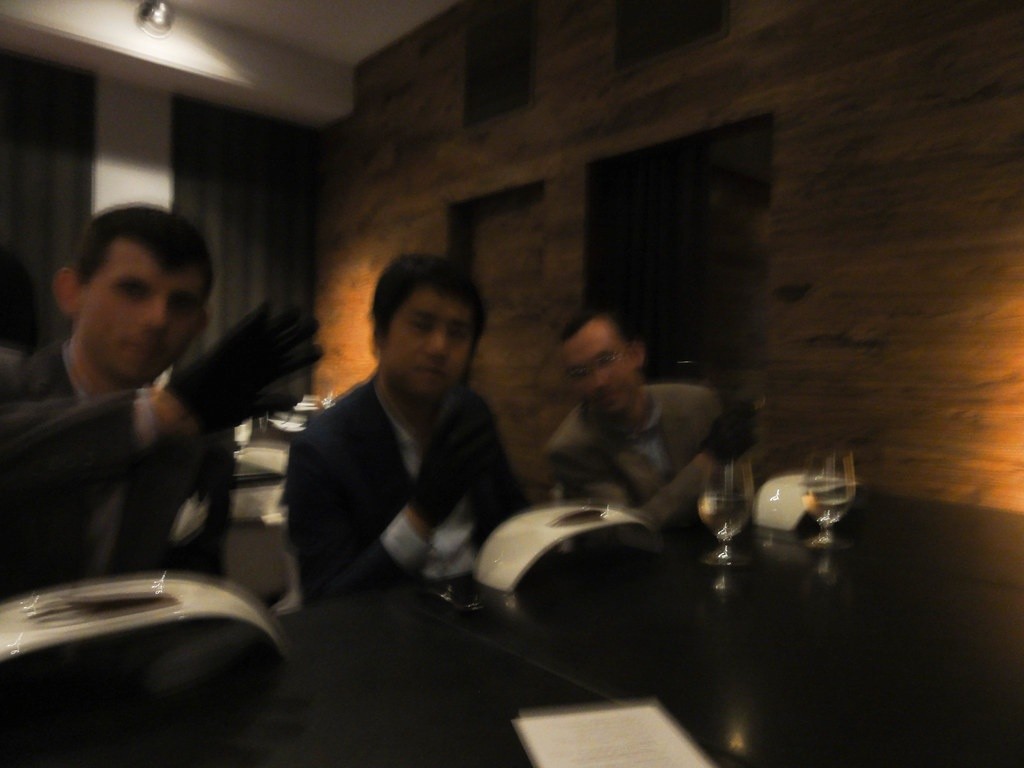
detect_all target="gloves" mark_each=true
[163,300,324,439]
[701,403,762,465]
[407,391,494,528]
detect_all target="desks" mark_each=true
[1,495,1024,768]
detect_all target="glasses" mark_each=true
[560,350,626,380]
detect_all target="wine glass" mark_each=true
[803,448,856,546]
[697,453,755,565]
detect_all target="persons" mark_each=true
[286,254,539,600]
[0,204,325,603]
[547,310,764,532]
